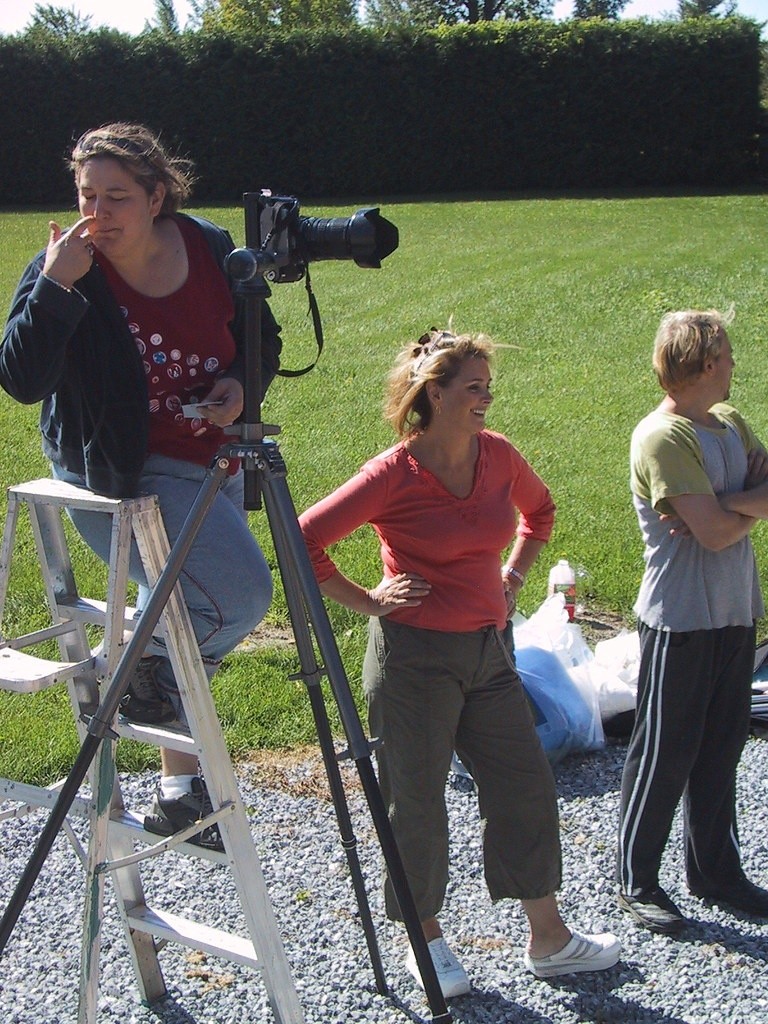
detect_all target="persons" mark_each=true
[299,327,621,997]
[617,306,768,931]
[0,123,284,852]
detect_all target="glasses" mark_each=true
[417,332,458,370]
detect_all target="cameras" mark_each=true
[260,197,399,284]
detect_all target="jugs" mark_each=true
[568,563,593,616]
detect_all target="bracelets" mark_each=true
[503,565,525,585]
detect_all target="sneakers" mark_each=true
[117,652,177,724]
[524,931,623,977]
[406,937,472,999]
[687,867,768,924]
[143,775,225,851]
[618,886,686,932]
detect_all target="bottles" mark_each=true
[548,560,575,622]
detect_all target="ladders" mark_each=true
[1,478,306,1024]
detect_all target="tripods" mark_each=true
[0,191,452,1024]
